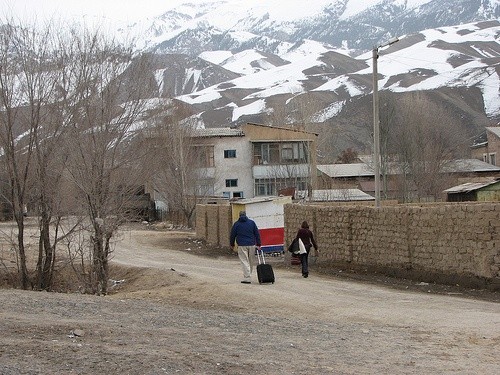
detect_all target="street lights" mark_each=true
[373,37,400,206]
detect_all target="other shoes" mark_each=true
[241,281,251,284]
[302,272,308,277]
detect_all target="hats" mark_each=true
[239,210,247,215]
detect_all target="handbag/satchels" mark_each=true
[291,254,300,265]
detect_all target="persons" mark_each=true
[229,209,262,284]
[284,221,320,278]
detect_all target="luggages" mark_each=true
[256,249,275,284]
